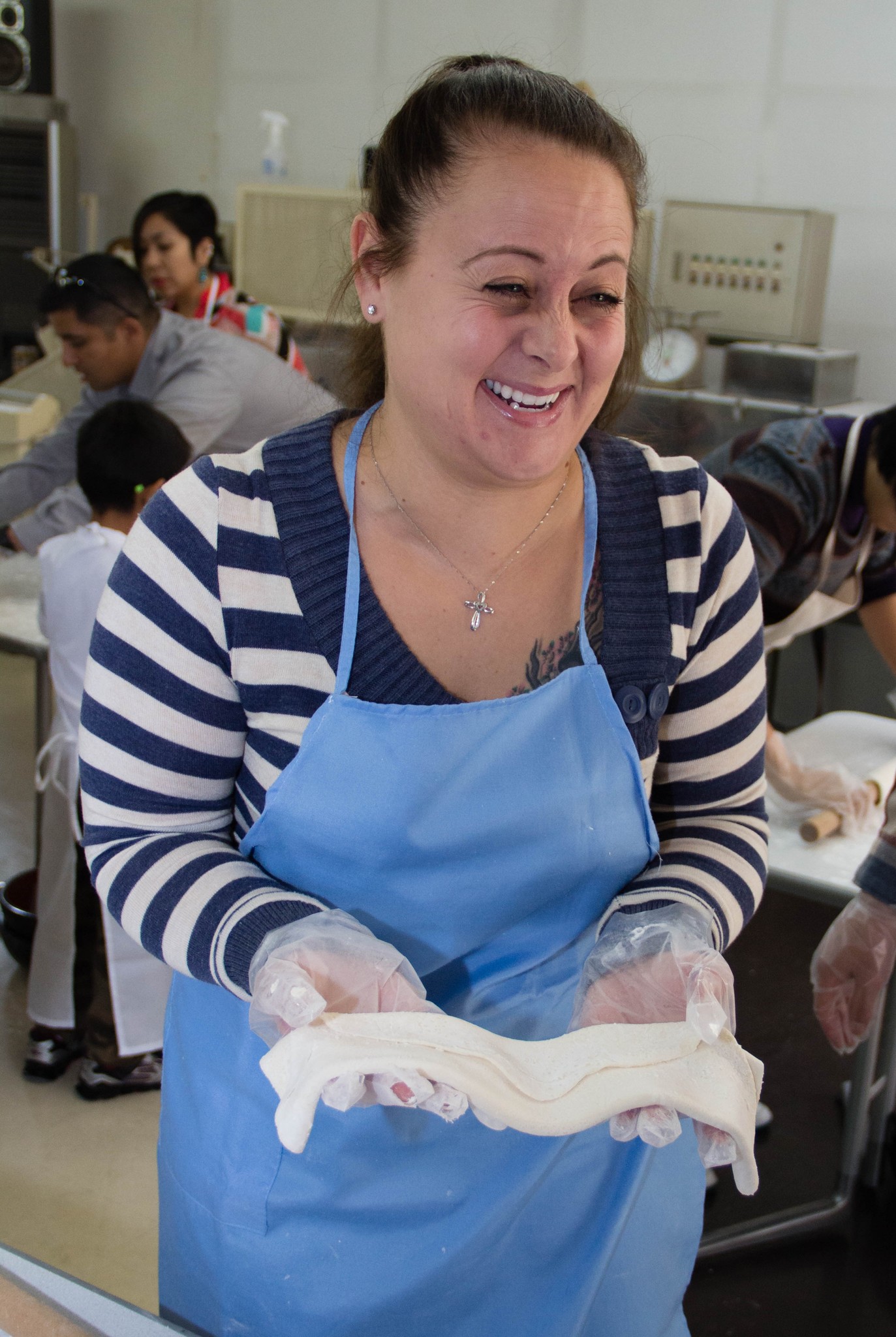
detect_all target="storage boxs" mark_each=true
[722,342,859,408]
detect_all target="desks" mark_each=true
[699,710,896,1255]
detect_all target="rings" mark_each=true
[0,526,19,560]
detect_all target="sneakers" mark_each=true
[77,1053,163,1101]
[22,1025,85,1080]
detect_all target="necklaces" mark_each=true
[370,409,569,633]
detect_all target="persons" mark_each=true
[130,190,310,378]
[702,407,896,1190]
[812,775,896,1055]
[75,58,774,1337]
[0,250,344,559]
[23,394,201,1102]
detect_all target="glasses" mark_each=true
[48,267,140,319]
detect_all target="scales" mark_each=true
[638,301,724,389]
